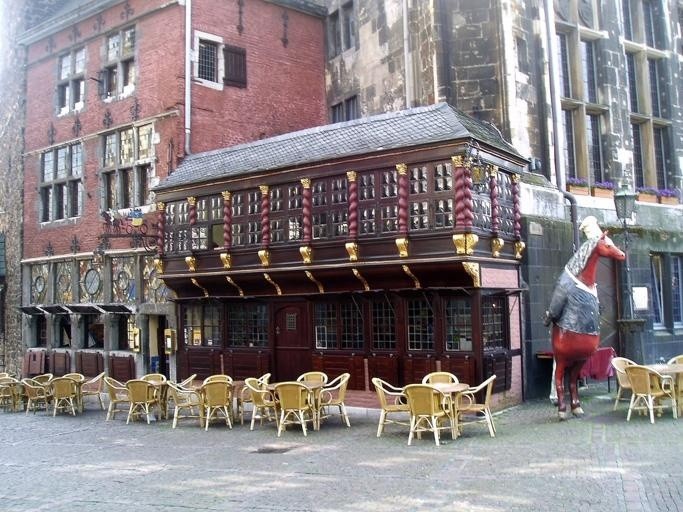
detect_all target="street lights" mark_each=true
[611,170,650,403]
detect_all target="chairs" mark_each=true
[609,354,682,424]
[199,379,234,432]
[315,371,352,432]
[421,371,458,385]
[140,372,165,380]
[273,381,318,438]
[202,373,233,385]
[1,365,85,417]
[370,376,417,439]
[403,383,457,447]
[167,372,198,419]
[235,372,273,427]
[244,377,282,432]
[78,371,107,414]
[166,379,206,430]
[103,376,134,422]
[453,373,497,443]
[296,370,329,385]
[124,378,163,425]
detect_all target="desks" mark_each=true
[264,380,326,429]
[536,348,615,398]
[123,380,167,417]
[402,381,470,441]
